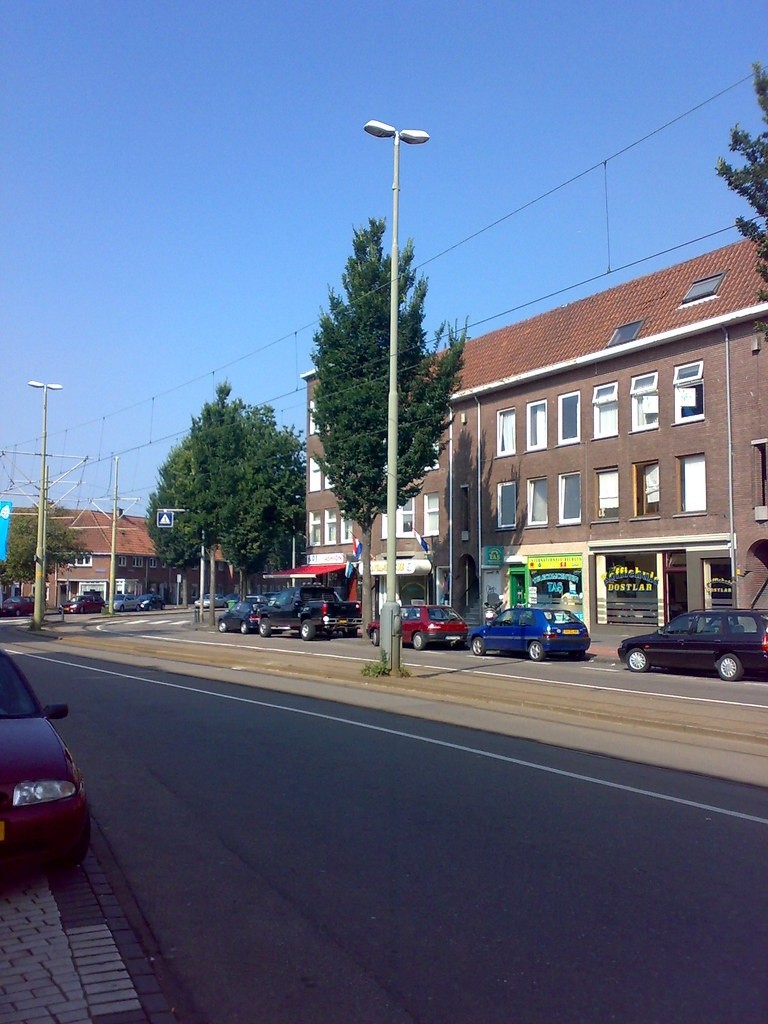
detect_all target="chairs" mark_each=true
[717,623,744,633]
[700,623,715,633]
[408,610,417,619]
[428,609,456,619]
[547,619,554,625]
[520,614,535,625]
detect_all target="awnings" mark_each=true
[263,564,346,578]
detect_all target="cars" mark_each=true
[59,594,106,615]
[0,646,91,875]
[466,607,591,662]
[105,594,140,612]
[0,596,34,617]
[137,593,166,611]
[366,604,469,651]
[194,593,228,608]
[216,590,281,634]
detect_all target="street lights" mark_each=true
[29,380,62,630]
[361,118,432,678]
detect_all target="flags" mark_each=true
[345,561,354,578]
[414,531,430,553]
[353,535,362,560]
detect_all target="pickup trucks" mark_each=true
[255,584,361,640]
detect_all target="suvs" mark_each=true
[616,608,768,684]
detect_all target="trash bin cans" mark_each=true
[227,599,239,609]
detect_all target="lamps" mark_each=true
[460,412,467,423]
[751,335,761,352]
